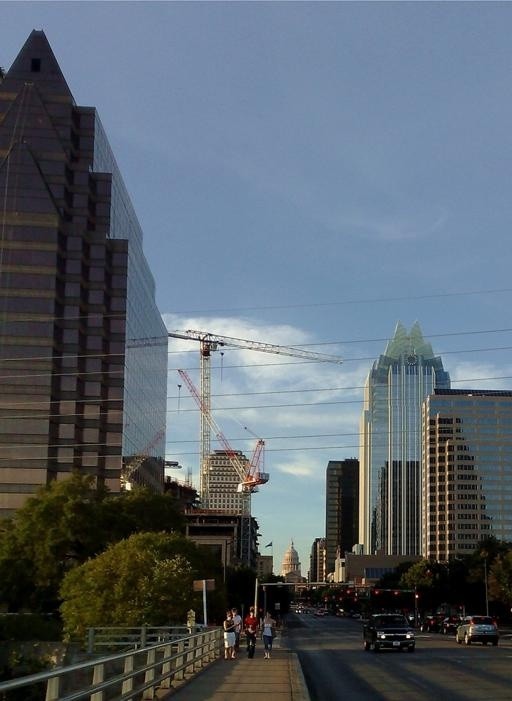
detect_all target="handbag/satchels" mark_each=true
[270,620,277,638]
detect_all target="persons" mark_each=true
[223,606,276,660]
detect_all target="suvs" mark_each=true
[455,615,499,646]
[362,613,415,652]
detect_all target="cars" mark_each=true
[296,603,420,622]
[437,616,463,636]
[419,615,444,632]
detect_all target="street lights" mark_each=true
[480,548,490,615]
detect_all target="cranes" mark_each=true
[165,326,345,507]
